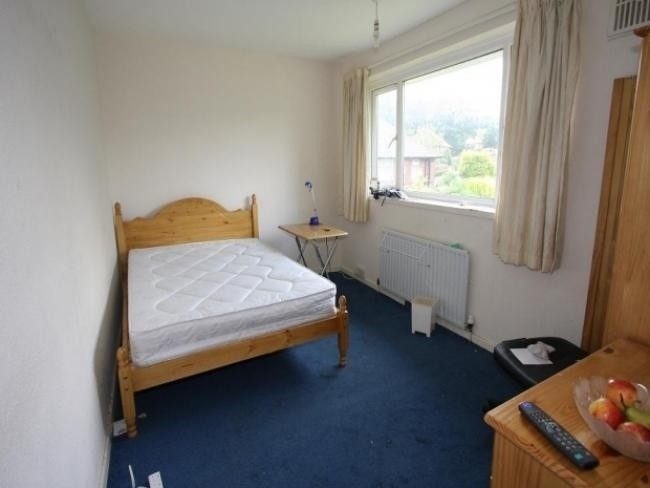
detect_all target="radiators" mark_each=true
[377,226,471,333]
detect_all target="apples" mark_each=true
[607,378,638,410]
[589,398,624,429]
[616,423,650,444]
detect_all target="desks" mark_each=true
[481,337,649,488]
[276,220,350,277]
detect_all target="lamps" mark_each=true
[305,180,322,227]
[371,1,382,49]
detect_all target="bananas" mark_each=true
[624,407,650,429]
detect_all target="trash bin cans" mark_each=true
[411,297,438,338]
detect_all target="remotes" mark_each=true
[519,400,599,471]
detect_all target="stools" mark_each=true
[492,336,585,383]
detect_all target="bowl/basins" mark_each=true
[574,375,650,464]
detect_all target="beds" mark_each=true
[113,193,348,440]
